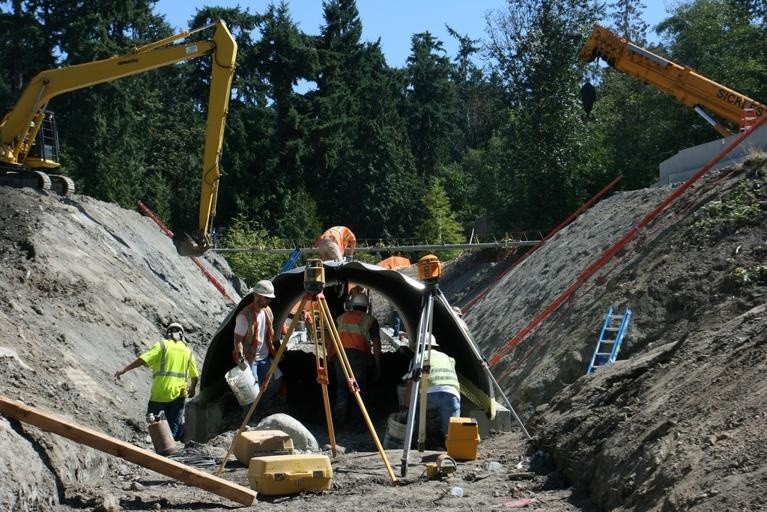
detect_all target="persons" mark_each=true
[317,226,355,260]
[406,332,461,439]
[113,322,199,440]
[391,307,400,337]
[302,309,314,344]
[233,280,284,426]
[327,294,381,425]
[348,286,365,300]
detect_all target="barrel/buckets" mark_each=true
[397,383,411,413]
[224,365,260,406]
[382,413,416,450]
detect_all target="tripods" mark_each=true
[400,285,533,478]
[212,289,397,481]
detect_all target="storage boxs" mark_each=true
[445,416,481,460]
[246,454,333,496]
[232,429,295,465]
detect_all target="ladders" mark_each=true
[586,307,632,376]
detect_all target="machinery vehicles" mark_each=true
[568,20,767,139]
[1,14,245,259]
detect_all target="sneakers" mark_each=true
[333,403,347,427]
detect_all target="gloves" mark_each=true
[346,255,353,262]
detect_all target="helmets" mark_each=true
[351,294,369,307]
[254,279,276,299]
[167,322,185,334]
[414,332,440,348]
